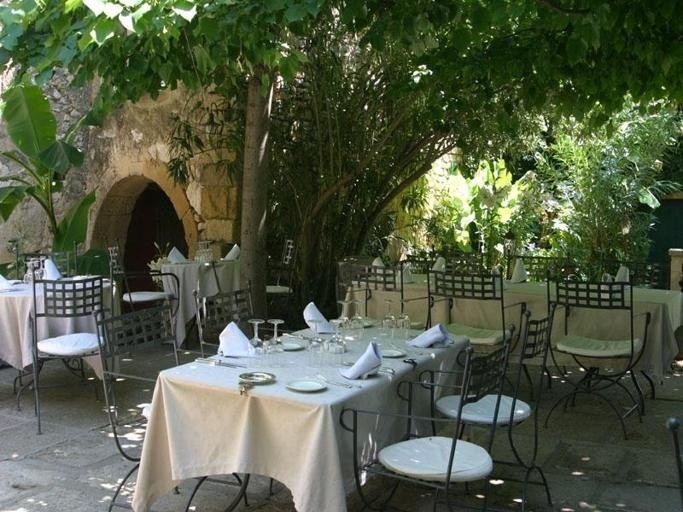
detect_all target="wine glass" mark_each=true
[22,256,43,285]
[246,300,409,372]
[194,241,215,265]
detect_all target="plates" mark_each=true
[377,347,405,358]
[241,371,275,386]
[349,321,371,328]
[280,339,307,350]
[288,378,326,392]
